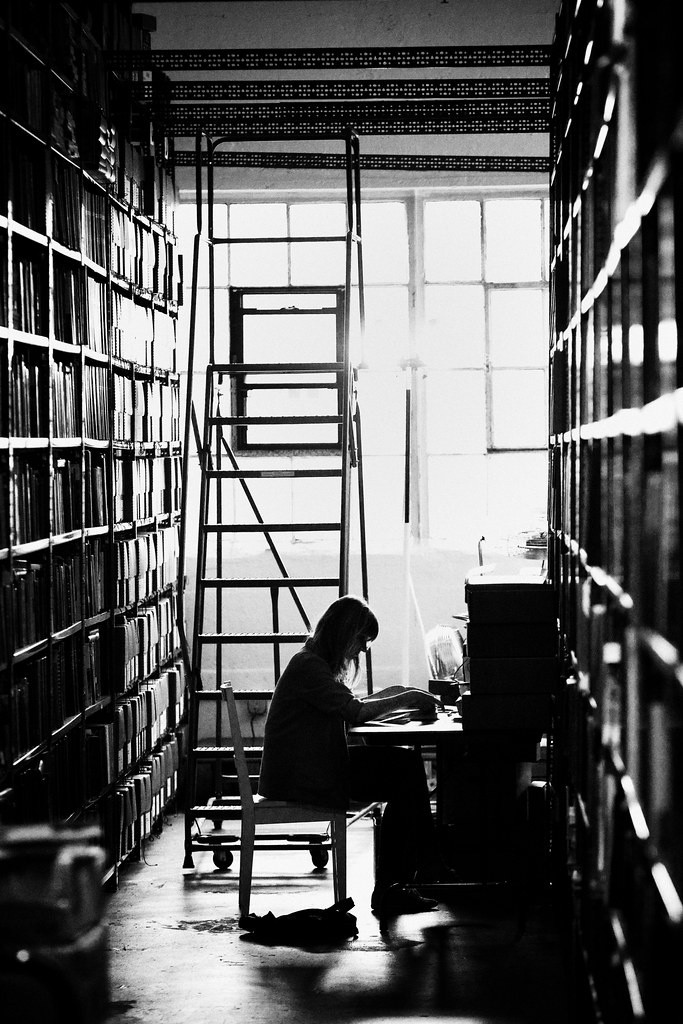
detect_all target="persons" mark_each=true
[258,596,438,916]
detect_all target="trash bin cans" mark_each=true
[1,822,110,1024]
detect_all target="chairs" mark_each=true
[216,681,352,925]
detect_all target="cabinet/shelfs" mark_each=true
[0,0,194,938]
[469,0,683,1024]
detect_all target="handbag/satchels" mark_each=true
[239,896,359,948]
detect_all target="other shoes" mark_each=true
[415,863,471,895]
[371,880,438,913]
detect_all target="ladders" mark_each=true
[180,361,350,869]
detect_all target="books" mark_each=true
[0,0,192,900]
[548,0,683,1024]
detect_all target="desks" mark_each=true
[348,712,537,925]
